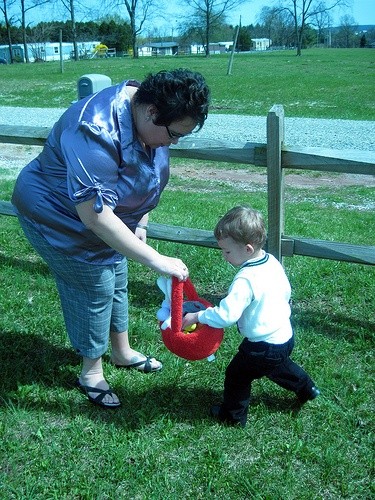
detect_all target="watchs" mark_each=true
[136,223,147,230]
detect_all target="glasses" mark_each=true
[165,126,184,141]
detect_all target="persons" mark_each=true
[182,206,319,429]
[10,66,212,407]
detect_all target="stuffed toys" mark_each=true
[156,276,206,334]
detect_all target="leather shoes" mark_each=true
[296,385,321,404]
[208,406,246,428]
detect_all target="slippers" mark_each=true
[115,356,164,373]
[76,379,122,409]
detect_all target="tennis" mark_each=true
[183,323,196,335]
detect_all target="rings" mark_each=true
[183,267,187,271]
